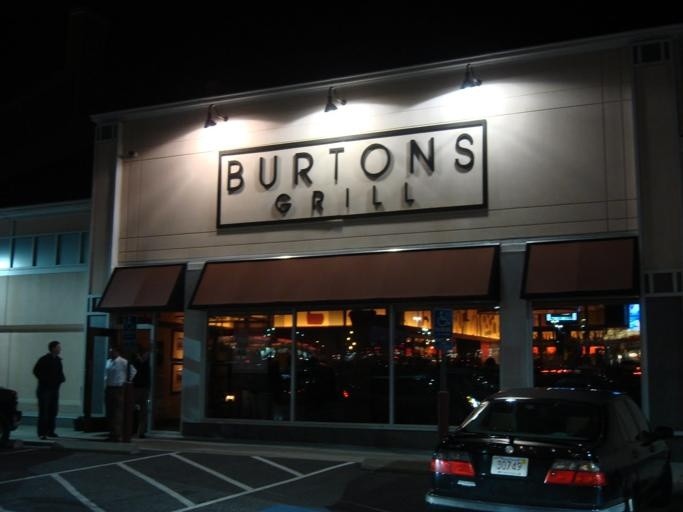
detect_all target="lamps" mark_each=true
[321,83,348,118]
[201,103,231,131]
[459,64,490,95]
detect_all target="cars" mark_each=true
[426,385,675,512]
[0,385,23,449]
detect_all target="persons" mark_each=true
[30,341,65,440]
[128,346,151,439]
[101,344,137,442]
